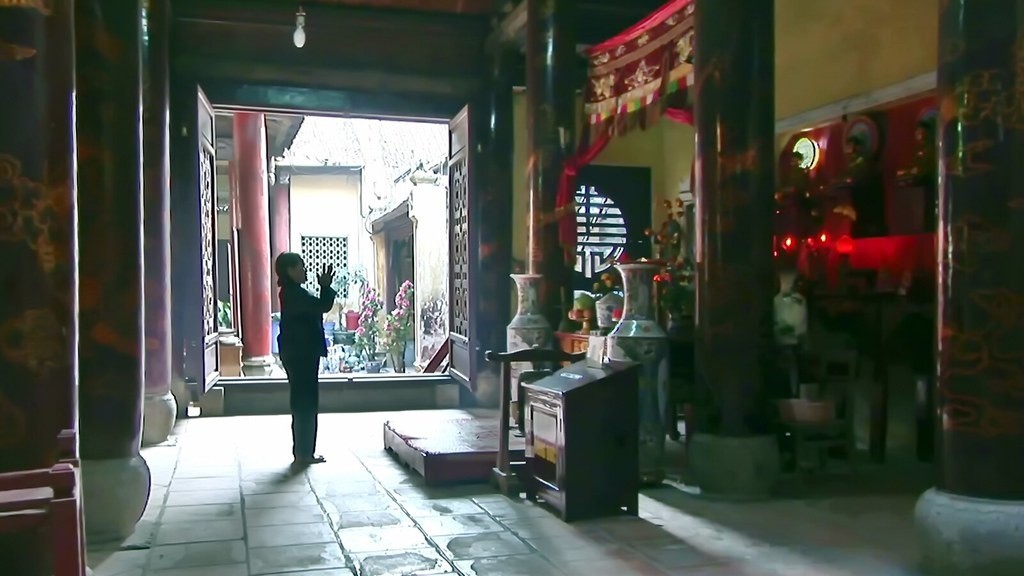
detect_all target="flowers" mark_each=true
[353,281,384,356]
[381,281,414,350]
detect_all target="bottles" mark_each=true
[606,264,670,488]
[505,274,554,402]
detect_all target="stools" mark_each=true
[769,418,846,498]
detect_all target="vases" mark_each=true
[605,263,670,489]
[388,340,406,374]
[506,273,553,438]
[364,361,382,373]
[322,322,335,335]
[346,312,359,330]
[333,330,356,346]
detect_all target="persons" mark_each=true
[275,251,337,463]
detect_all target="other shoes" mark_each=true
[295,456,322,462]
